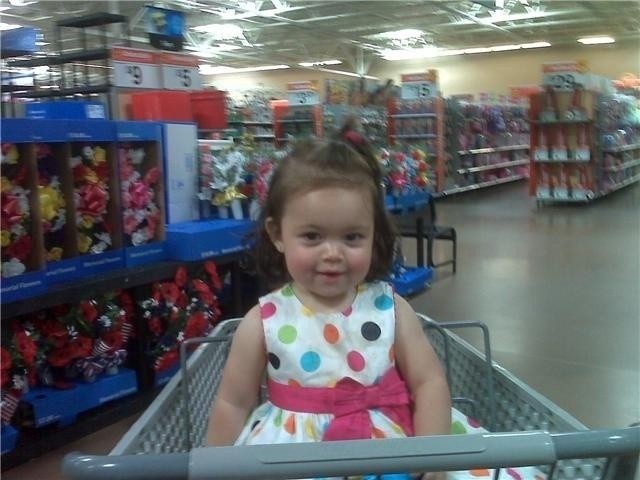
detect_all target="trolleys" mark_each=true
[61,313,639,480]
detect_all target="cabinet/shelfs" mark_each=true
[2,4,640,480]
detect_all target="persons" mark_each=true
[199,114,537,480]
